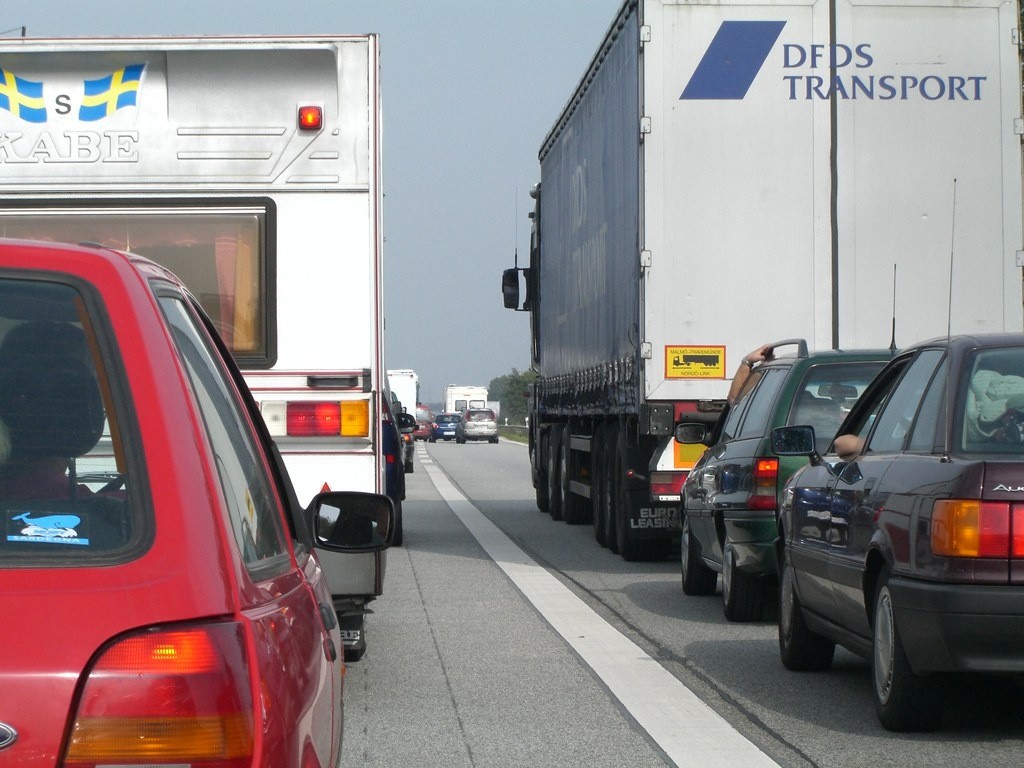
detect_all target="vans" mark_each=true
[411,405,435,442]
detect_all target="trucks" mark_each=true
[444,384,489,414]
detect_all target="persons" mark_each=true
[0,320,126,507]
[370,499,387,536]
[503,273,519,294]
[833,434,864,457]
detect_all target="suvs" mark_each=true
[675,339,907,621]
[455,407,498,444]
[767,332,1022,733]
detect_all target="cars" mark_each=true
[0,234,396,768]
[381,389,416,547]
[432,412,461,442]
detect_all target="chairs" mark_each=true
[799,398,842,438]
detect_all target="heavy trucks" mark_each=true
[0,31,390,665]
[385,369,419,473]
[501,0,1022,563]
